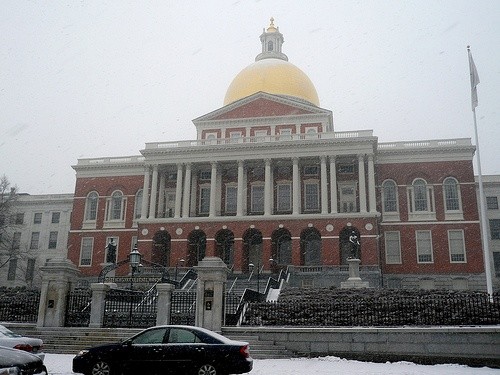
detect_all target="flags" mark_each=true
[469,51,480,111]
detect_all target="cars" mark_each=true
[0,346,48,375]
[0,324,45,362]
[73,324,253,375]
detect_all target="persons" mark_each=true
[104,237,117,264]
[348,231,360,259]
